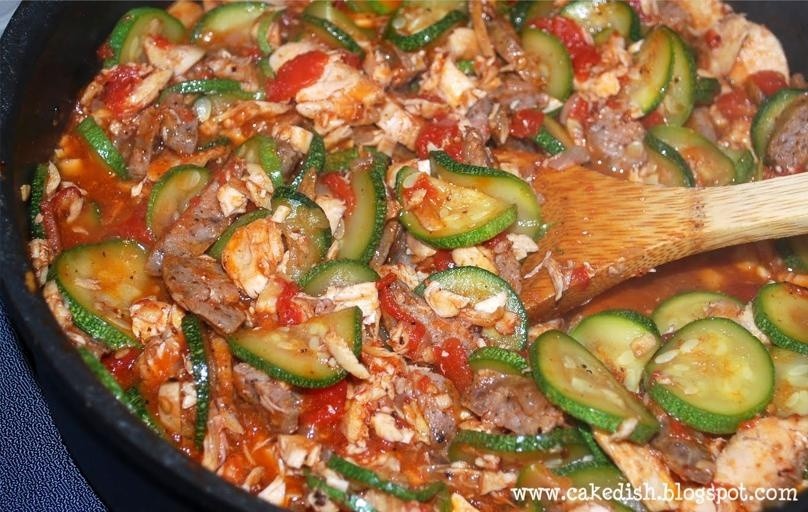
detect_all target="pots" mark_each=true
[1,0,808,512]
